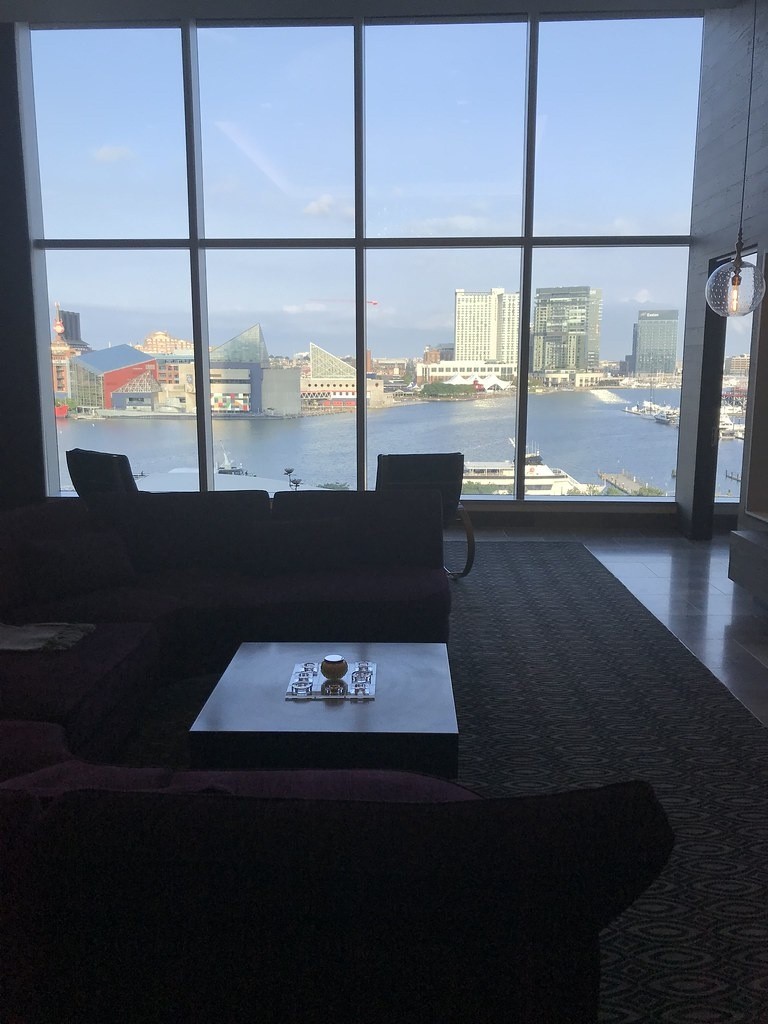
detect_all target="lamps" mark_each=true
[704,0,765,318]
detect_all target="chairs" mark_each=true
[66,449,138,498]
[375,453,475,580]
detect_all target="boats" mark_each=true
[639,410,680,428]
[168,439,256,477]
[460,437,606,496]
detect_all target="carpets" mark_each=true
[116,540,768,1024]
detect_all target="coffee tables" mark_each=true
[188,643,459,782]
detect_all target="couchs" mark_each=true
[0,490,675,1024]
